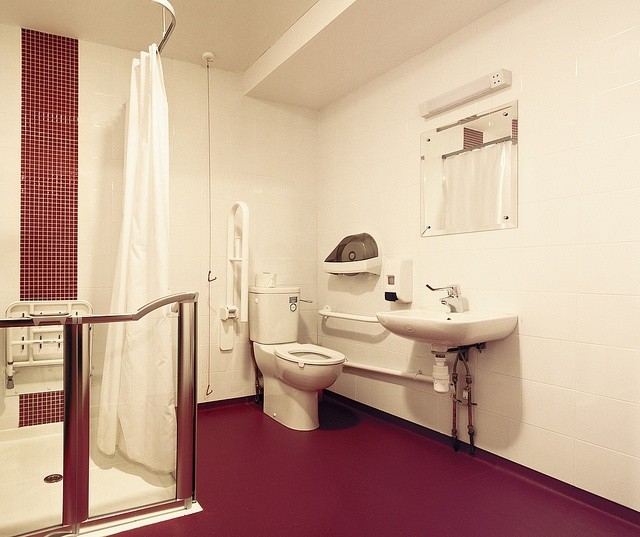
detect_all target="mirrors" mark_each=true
[417,101,519,240]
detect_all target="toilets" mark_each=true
[248,287,346,431]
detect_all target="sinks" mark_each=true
[376,309,518,353]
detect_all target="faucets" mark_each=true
[426,284,464,313]
